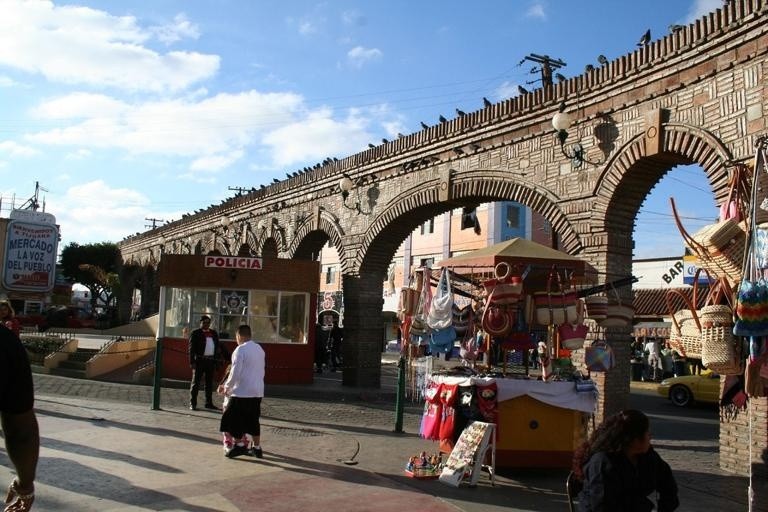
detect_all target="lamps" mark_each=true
[158,234,166,253]
[550,86,584,159]
[339,172,360,211]
[220,213,231,232]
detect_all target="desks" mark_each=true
[429,368,596,475]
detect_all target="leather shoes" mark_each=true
[205,403,217,409]
[190,405,196,410]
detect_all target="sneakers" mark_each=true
[223,439,262,458]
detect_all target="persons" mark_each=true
[315,322,343,373]
[526,333,547,369]
[217,324,265,459]
[630,336,682,381]
[188,315,220,410]
[0,298,39,512]
[573,409,680,512]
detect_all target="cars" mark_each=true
[657,368,720,407]
[14,305,122,330]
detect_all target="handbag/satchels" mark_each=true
[398,264,637,377]
[669,147,768,380]
